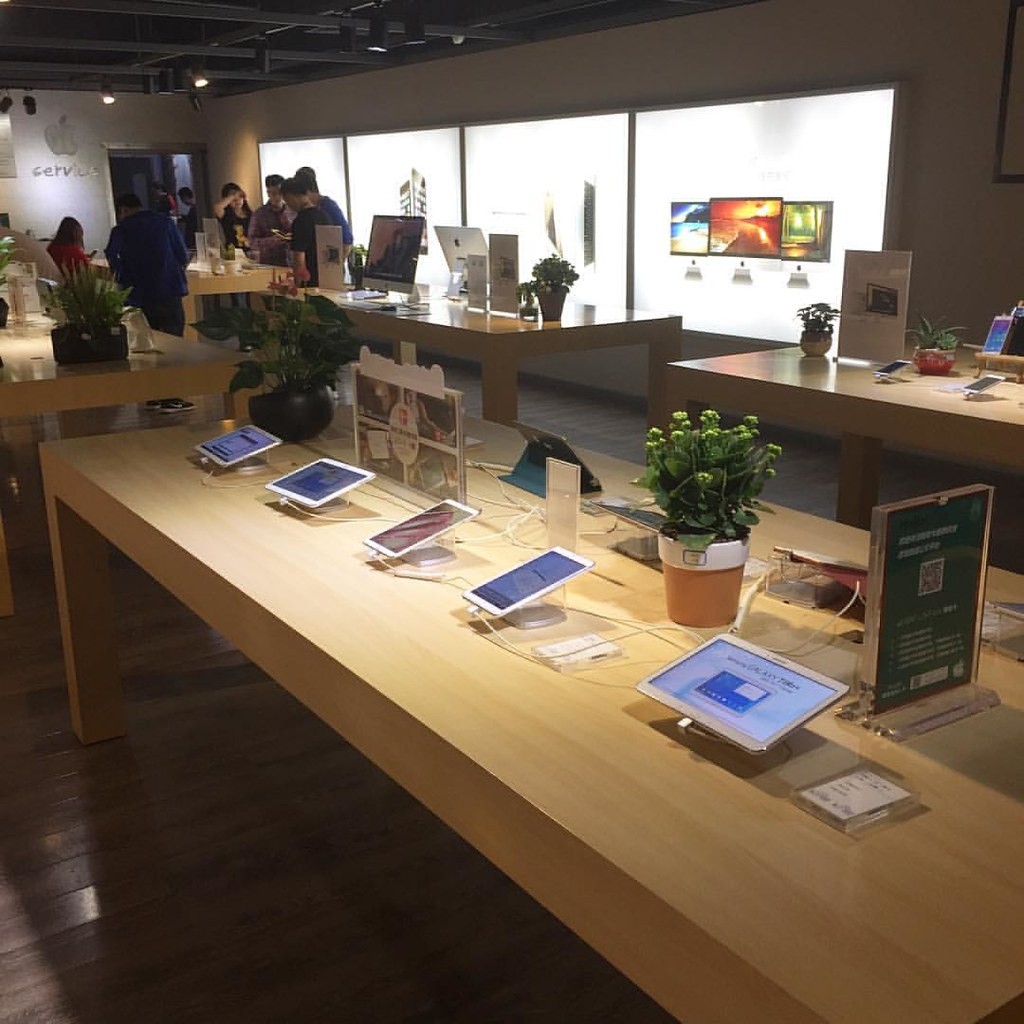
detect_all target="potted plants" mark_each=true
[794,302,843,355]
[512,280,540,323]
[905,311,972,372]
[1,233,26,329]
[624,406,784,630]
[347,242,373,291]
[188,262,368,450]
[43,264,136,365]
[525,253,579,323]
[216,238,242,277]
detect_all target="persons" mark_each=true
[378,228,405,272]
[49,217,93,276]
[215,183,252,257]
[247,167,354,289]
[104,194,189,337]
[177,187,199,250]
[151,181,177,221]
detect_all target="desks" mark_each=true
[659,337,1024,581]
[32,402,1023,1023]
[250,274,689,463]
[0,287,263,620]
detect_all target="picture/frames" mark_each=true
[985,0,1024,188]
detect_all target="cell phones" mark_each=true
[876,359,910,377]
[89,249,98,257]
[985,312,1012,355]
[964,373,1005,394]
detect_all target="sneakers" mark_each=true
[160,400,196,414]
[143,400,163,410]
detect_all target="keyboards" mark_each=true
[342,298,381,312]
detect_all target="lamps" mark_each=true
[0,87,15,116]
[23,87,38,116]
[101,78,116,105]
[188,64,208,89]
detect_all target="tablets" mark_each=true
[462,545,596,618]
[513,420,594,479]
[193,424,281,469]
[775,546,868,575]
[988,600,1024,619]
[363,496,479,556]
[263,454,376,511]
[590,501,664,534]
[637,631,849,758]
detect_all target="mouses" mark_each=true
[380,305,396,311]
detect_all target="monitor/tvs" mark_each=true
[778,200,834,262]
[358,212,427,291]
[668,201,710,257]
[706,195,785,260]
[435,225,492,287]
